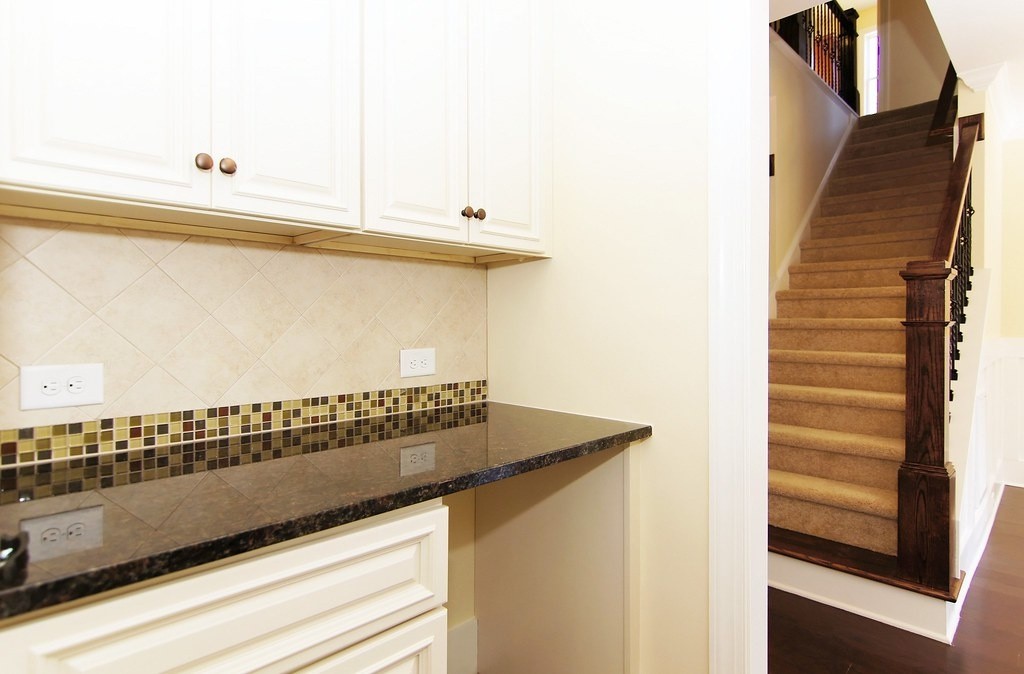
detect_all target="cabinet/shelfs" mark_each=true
[0,0,553,272]
[0,494,449,674]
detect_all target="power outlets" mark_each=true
[20,363,105,411]
[400,443,436,477]
[400,348,436,378]
[20,505,104,563]
